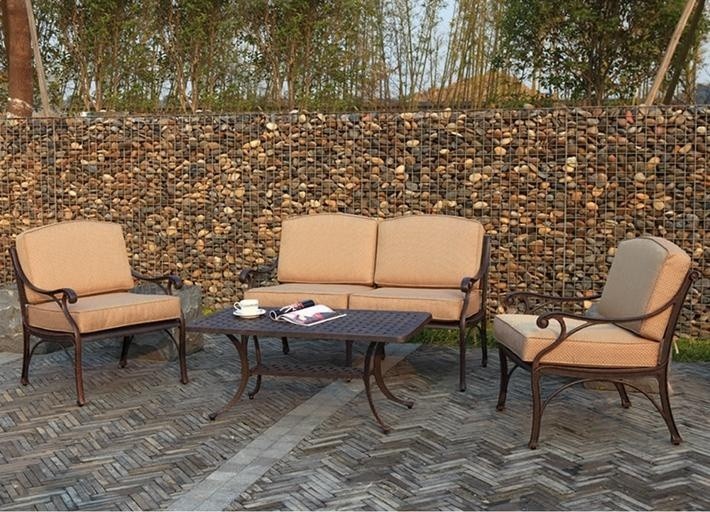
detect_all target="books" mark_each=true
[269,299,347,327]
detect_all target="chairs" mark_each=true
[494,237,694,450]
[8,220,189,406]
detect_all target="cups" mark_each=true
[234,299,258,314]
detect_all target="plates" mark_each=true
[234,308,265,319]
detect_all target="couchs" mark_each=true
[238,213,492,392]
